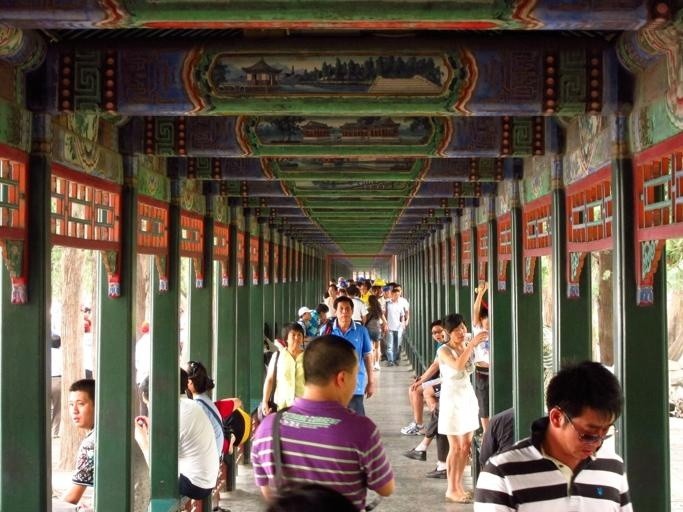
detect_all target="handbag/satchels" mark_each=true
[256,398,278,424]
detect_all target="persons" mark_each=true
[50,307,223,511]
[401,282,513,505]
[251,276,410,511]
[474,361,634,511]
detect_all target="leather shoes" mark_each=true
[403,447,426,462]
[424,469,446,480]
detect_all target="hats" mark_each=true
[295,307,314,316]
[336,282,348,290]
[140,322,150,333]
[372,278,386,287]
[382,286,390,291]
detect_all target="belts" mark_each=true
[52,375,62,380]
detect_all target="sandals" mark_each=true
[444,489,472,505]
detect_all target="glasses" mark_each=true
[556,405,619,444]
[431,329,443,336]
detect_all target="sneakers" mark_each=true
[373,364,380,373]
[398,423,424,437]
[394,361,400,367]
[386,360,392,368]
